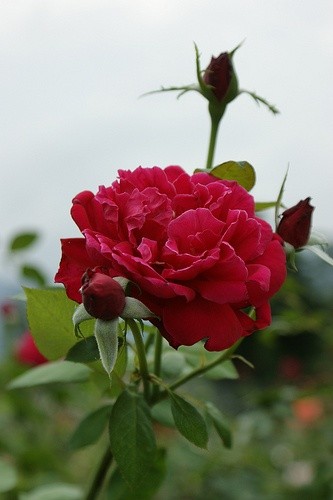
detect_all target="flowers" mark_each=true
[50,40,316,499]
